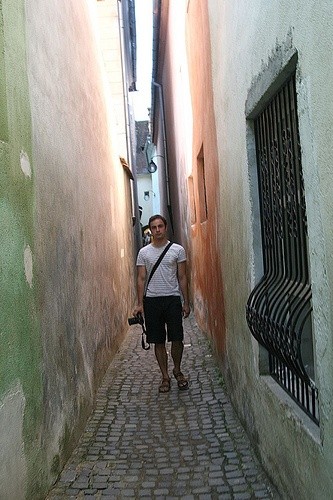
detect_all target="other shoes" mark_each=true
[159,377,172,393]
[173,371,189,389]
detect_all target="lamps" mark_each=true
[148,155,165,173]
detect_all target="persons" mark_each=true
[132,213,191,393]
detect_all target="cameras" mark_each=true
[127,311,144,325]
